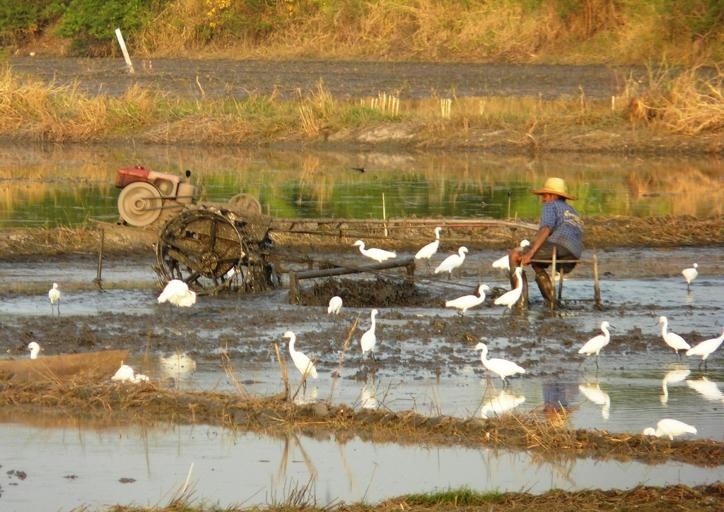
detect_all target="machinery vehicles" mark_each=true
[109,163,607,315]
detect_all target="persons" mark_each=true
[508,176,584,313]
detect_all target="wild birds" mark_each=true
[656,364,691,404]
[685,332,724,369]
[479,386,526,422]
[680,262,699,290]
[26,341,40,360]
[578,370,612,423]
[577,319,616,370]
[360,309,380,360]
[685,371,724,403]
[472,341,527,388]
[282,330,319,395]
[157,279,197,308]
[108,359,150,384]
[154,349,197,368]
[642,418,698,442]
[361,372,380,411]
[327,295,342,315]
[656,315,691,360]
[349,225,533,319]
[47,281,60,305]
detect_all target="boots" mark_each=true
[509,267,530,312]
[534,270,559,310]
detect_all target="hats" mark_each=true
[529,176,577,201]
